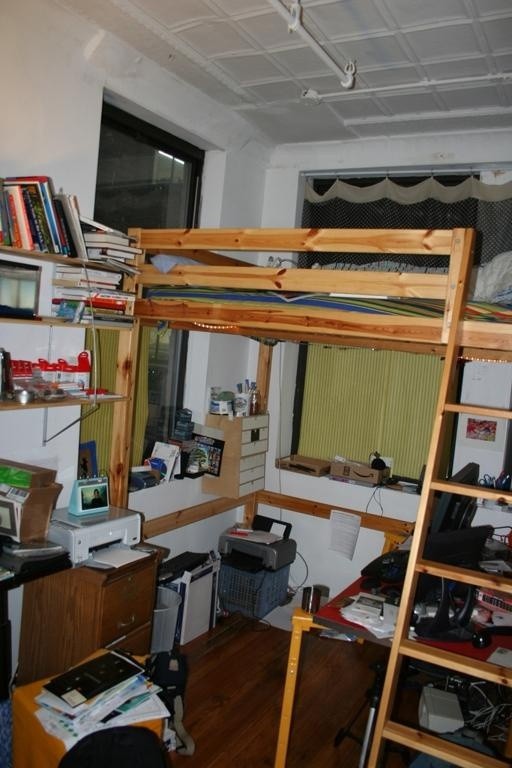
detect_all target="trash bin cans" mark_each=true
[151,586,182,654]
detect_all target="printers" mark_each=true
[47,504,157,570]
[217,514,297,573]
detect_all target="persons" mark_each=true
[80,455,90,478]
[90,488,107,508]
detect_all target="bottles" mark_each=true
[232,382,261,418]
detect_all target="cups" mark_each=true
[302,587,321,616]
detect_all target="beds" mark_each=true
[110,223,510,768]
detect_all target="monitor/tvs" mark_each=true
[430,460,479,530]
[413,524,494,641]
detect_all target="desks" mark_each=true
[272,571,512,768]
[0,528,126,704]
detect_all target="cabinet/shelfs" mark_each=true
[14,542,170,685]
[0,243,133,410]
[202,412,270,498]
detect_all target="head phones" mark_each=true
[472,621,512,648]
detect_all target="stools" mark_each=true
[9,672,167,766]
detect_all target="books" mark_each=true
[0,173,145,331]
[340,591,400,638]
[32,646,174,753]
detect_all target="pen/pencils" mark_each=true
[237,379,257,399]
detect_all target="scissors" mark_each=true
[479,474,492,488]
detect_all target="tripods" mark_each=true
[331,660,411,768]
